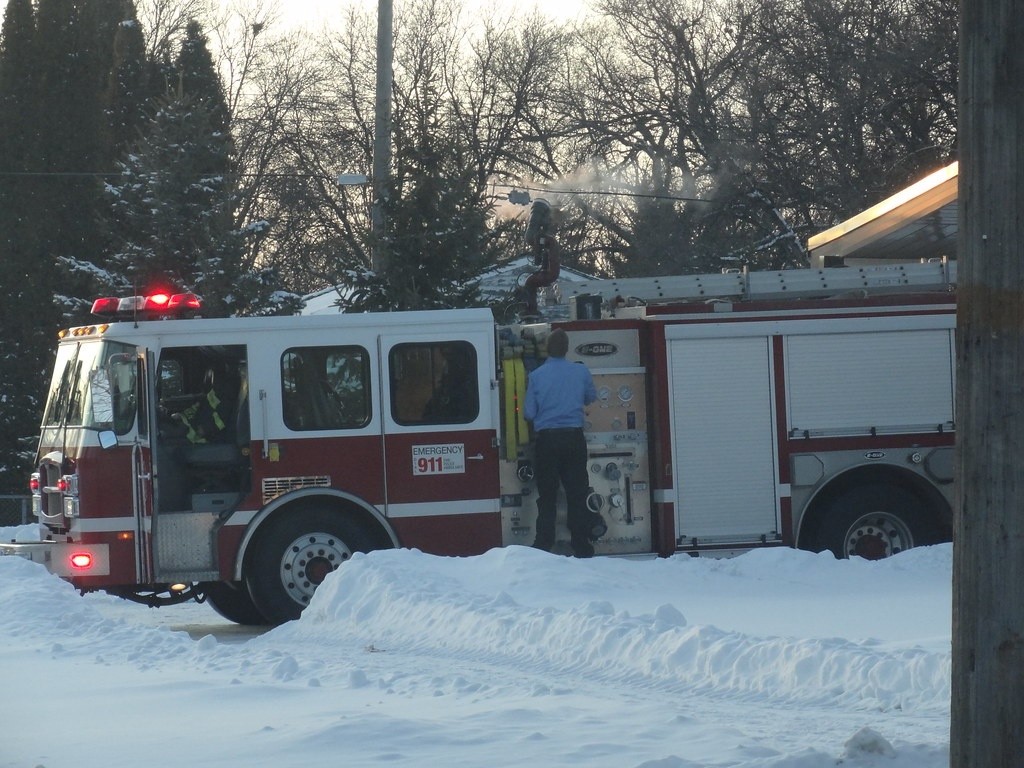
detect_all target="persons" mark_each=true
[523,329,597,558]
[171,387,233,443]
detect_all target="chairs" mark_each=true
[182,361,251,493]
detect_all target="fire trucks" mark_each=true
[30,198,959,629]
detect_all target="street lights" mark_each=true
[334,174,396,284]
